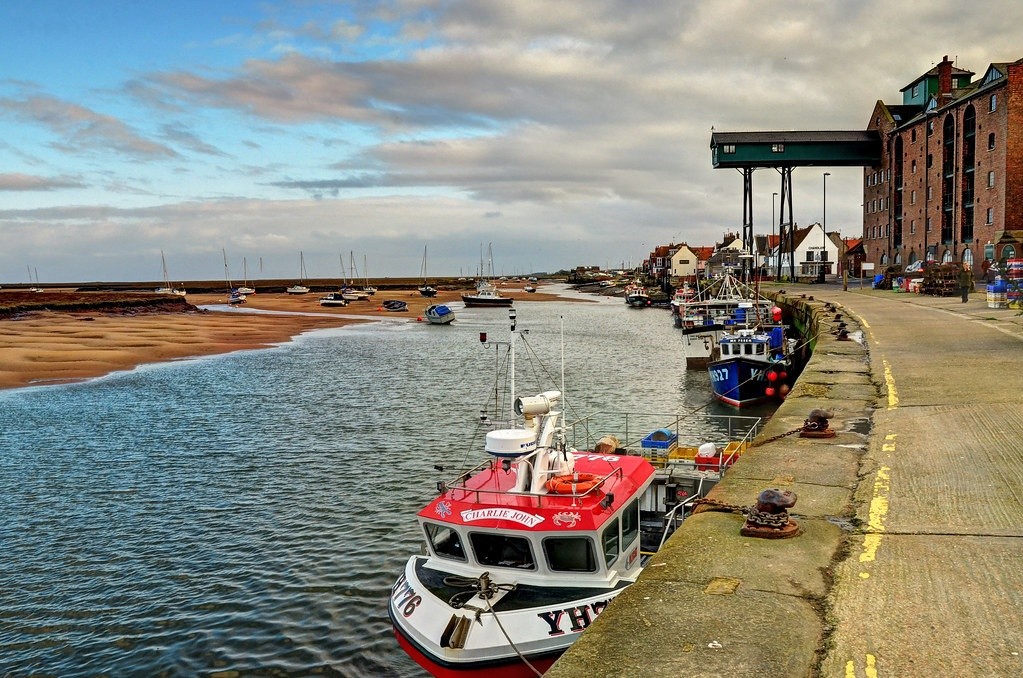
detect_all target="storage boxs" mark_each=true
[684,308,767,324]
[641,433,751,472]
[987,258,1023,309]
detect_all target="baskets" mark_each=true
[986,280,1008,308]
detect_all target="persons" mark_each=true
[958,262,974,304]
[981,257,997,279]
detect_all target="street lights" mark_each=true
[773,192,778,281]
[824,173,830,271]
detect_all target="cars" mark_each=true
[905,260,923,273]
[499,277,537,281]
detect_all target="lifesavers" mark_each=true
[544,472,605,494]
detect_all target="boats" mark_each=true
[461,282,514,307]
[599,281,616,287]
[629,287,649,308]
[230,289,247,303]
[382,300,407,311]
[671,274,797,370]
[524,284,536,292]
[319,292,349,307]
[706,327,793,411]
[387,308,655,678]
[425,304,455,324]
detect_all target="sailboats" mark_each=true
[27,264,43,293]
[238,257,255,294]
[286,251,310,293]
[339,251,377,300]
[154,250,186,297]
